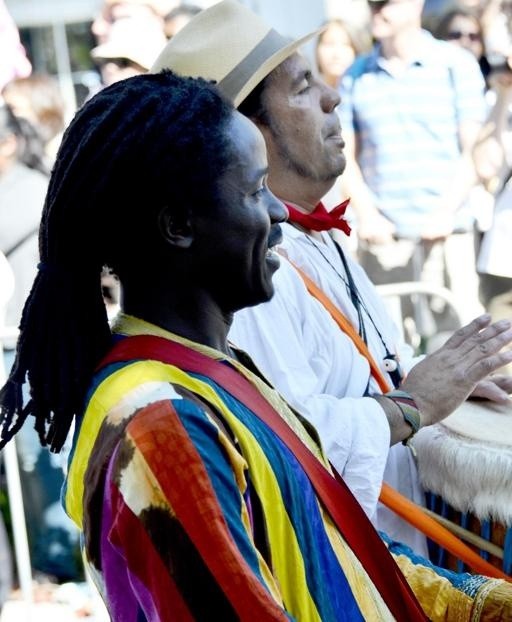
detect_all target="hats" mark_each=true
[144,0,326,111]
[90,15,166,70]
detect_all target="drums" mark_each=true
[417,397,511,578]
